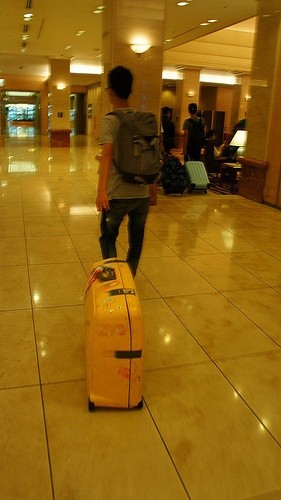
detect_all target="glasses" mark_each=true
[105,87,111,92]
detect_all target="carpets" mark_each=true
[208,173,241,195]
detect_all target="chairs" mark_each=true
[200,139,228,175]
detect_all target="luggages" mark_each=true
[83,258,144,413]
[160,150,187,196]
[184,154,211,194]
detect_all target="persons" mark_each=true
[205,130,224,157]
[162,107,178,152]
[96,66,158,277]
[183,103,205,160]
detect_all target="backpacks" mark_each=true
[185,117,206,149]
[102,110,160,185]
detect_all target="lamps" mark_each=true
[130,43,153,57]
[229,130,247,163]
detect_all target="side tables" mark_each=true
[218,163,243,192]
